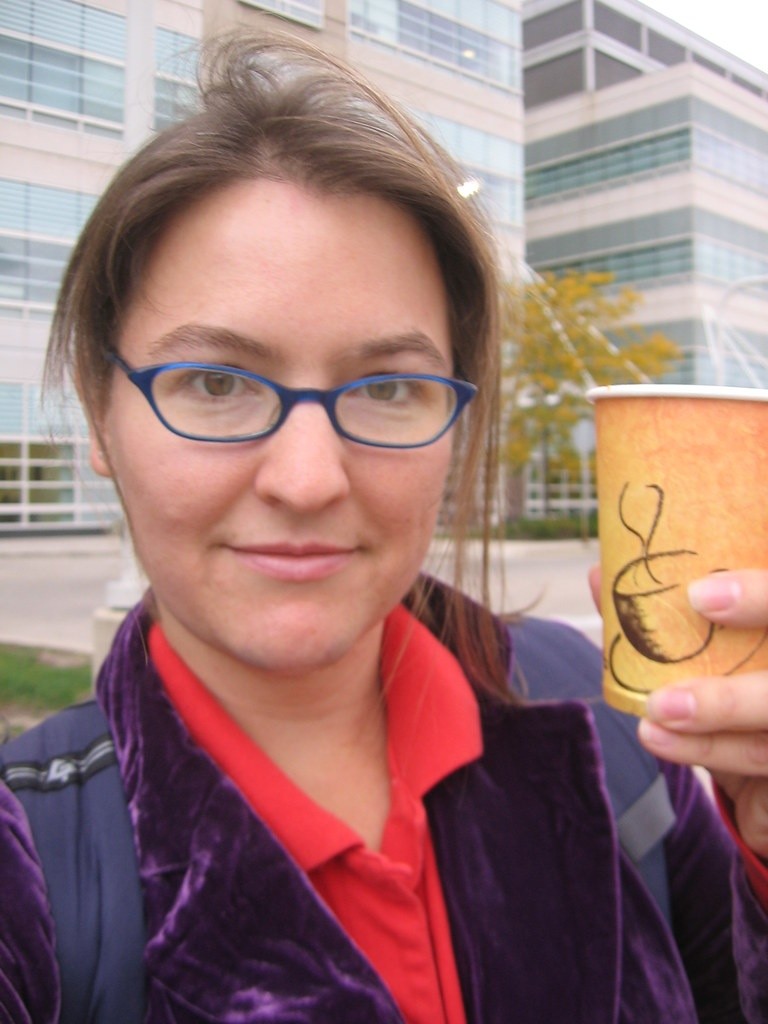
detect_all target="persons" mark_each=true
[0,30,768,1024]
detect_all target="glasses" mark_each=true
[107,348,479,448]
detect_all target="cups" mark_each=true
[584,382,768,720]
